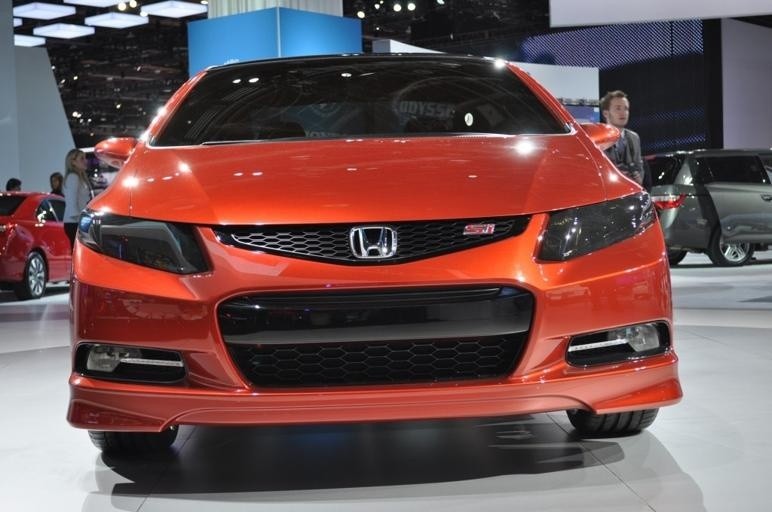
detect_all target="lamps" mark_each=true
[13,1,209,49]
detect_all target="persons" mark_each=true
[43,172,67,223]
[59,150,94,246]
[590,90,647,186]
[6,177,22,193]
